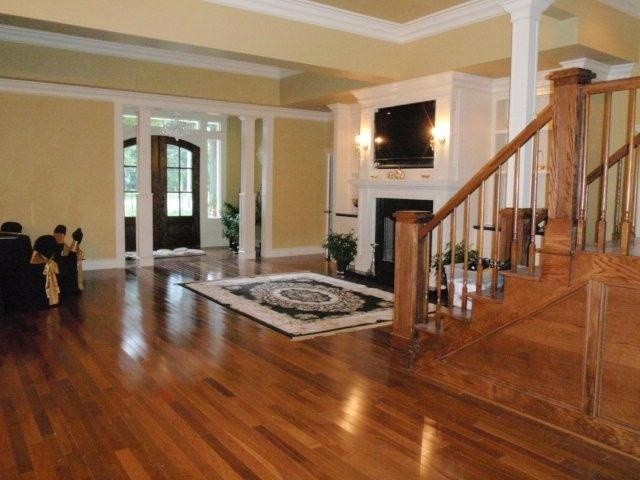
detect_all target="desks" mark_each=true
[0,232,32,315]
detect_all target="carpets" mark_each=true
[175,270,446,341]
[125,246,207,261]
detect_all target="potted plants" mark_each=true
[431,242,478,301]
[221,202,239,253]
[323,234,358,278]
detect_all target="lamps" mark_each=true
[355,135,368,151]
[429,127,445,150]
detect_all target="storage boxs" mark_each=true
[443,262,504,312]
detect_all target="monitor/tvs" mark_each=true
[373,100,436,170]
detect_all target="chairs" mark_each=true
[0,235,59,333]
[52,225,66,257]
[54,228,83,294]
[1,221,22,233]
